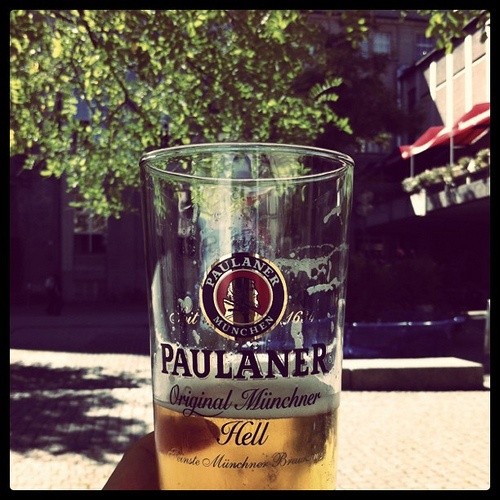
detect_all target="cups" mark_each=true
[141,137,358,490]
